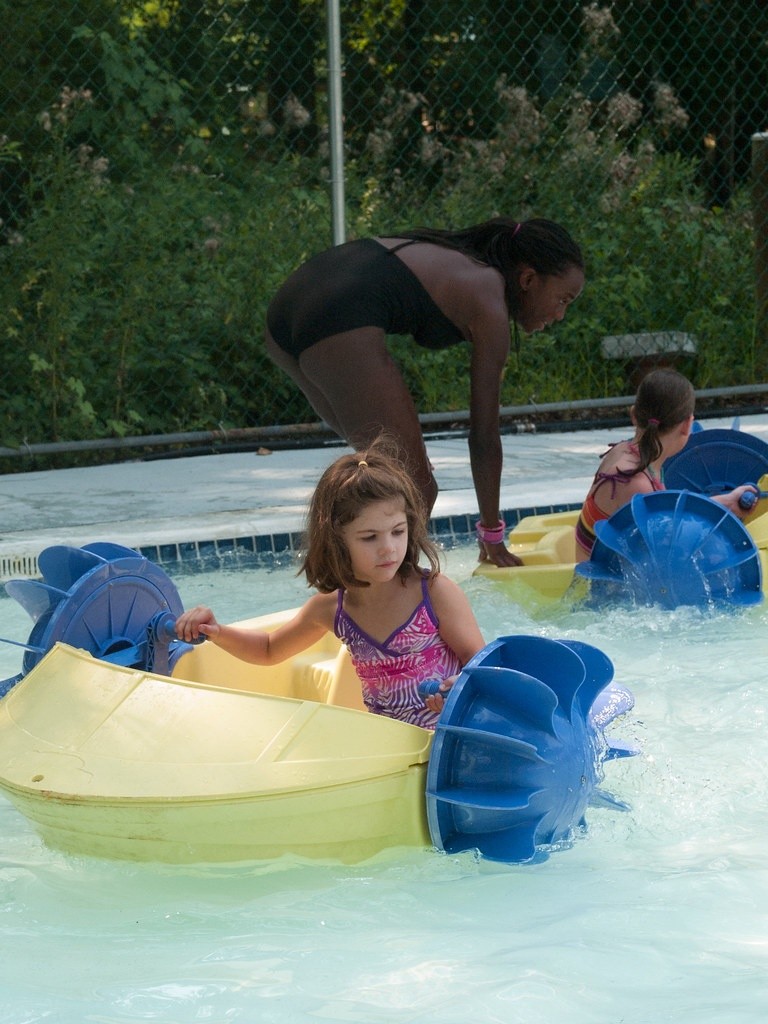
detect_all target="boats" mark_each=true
[1,542,642,864]
[470,429,768,616]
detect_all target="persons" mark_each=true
[175,449,488,736]
[575,367,758,565]
[264,217,594,566]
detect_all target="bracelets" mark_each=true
[476,520,505,544]
